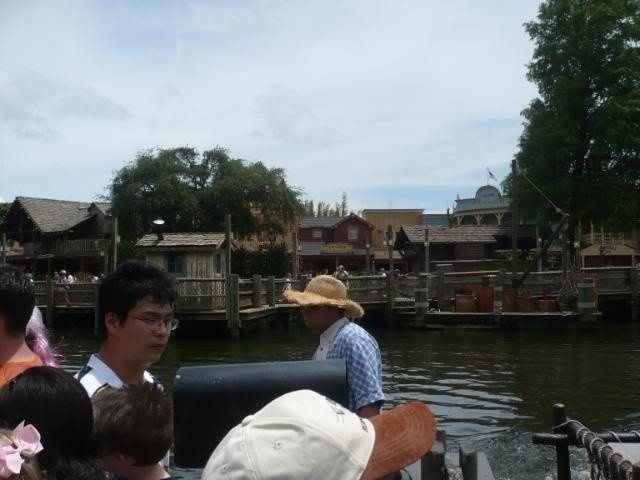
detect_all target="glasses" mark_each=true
[127,314,180,331]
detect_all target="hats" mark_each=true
[281,275,365,318]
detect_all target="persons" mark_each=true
[277,273,297,303]
[0,265,45,389]
[74,258,180,480]
[393,269,409,298]
[281,275,387,419]
[0,365,95,480]
[335,264,350,289]
[25,269,99,307]
[92,380,187,479]
[379,267,387,277]
